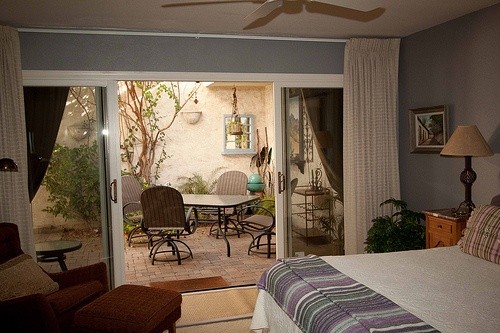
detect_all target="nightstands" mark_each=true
[423,207,473,249]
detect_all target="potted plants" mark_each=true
[319,193,345,255]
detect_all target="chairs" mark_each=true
[0,222,109,333]
[121,171,298,266]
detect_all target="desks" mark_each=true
[35,239,82,272]
[181,193,260,257]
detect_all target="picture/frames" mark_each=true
[407,104,449,154]
[289,92,304,161]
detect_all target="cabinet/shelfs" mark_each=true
[291,184,331,246]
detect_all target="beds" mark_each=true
[251,194,500,333]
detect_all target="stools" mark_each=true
[74,284,182,333]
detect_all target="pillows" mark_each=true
[459,205,500,265]
[0,254,59,301]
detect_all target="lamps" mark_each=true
[230,88,243,135]
[0,157,18,172]
[439,125,495,217]
